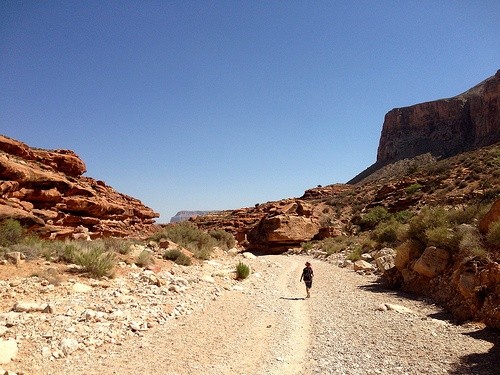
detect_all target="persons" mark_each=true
[300,262,314,298]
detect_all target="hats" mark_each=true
[305,261,312,266]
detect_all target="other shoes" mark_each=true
[307,291,310,298]
